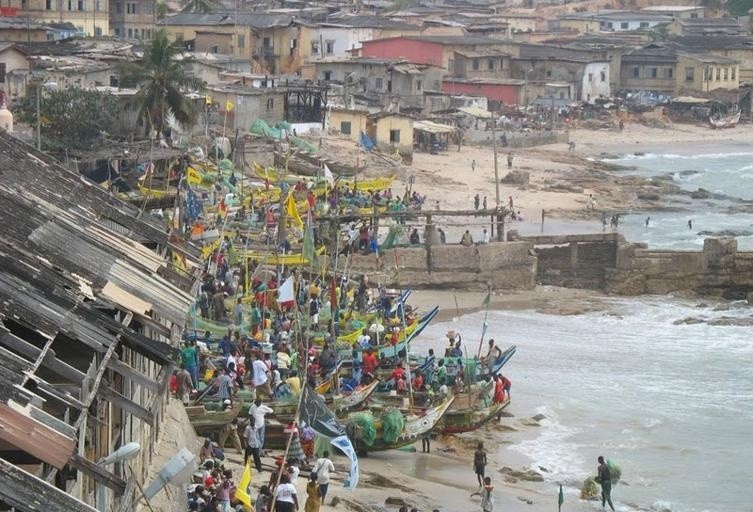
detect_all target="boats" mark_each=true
[114,145,426,247]
[173,246,518,456]
[706,103,743,129]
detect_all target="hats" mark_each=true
[224,399,231,404]
[194,471,203,478]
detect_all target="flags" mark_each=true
[205,92,213,106]
[225,99,234,113]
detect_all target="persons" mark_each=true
[567,140,575,151]
[510,211,516,220]
[584,193,597,211]
[610,216,617,228]
[600,212,610,226]
[187,438,335,511]
[500,132,508,147]
[506,151,513,168]
[616,213,623,227]
[516,211,522,220]
[473,442,486,487]
[243,416,263,472]
[593,456,616,512]
[480,477,494,511]
[506,196,513,210]
[148,172,511,455]
[644,216,650,227]
[472,160,475,171]
[686,219,692,229]
